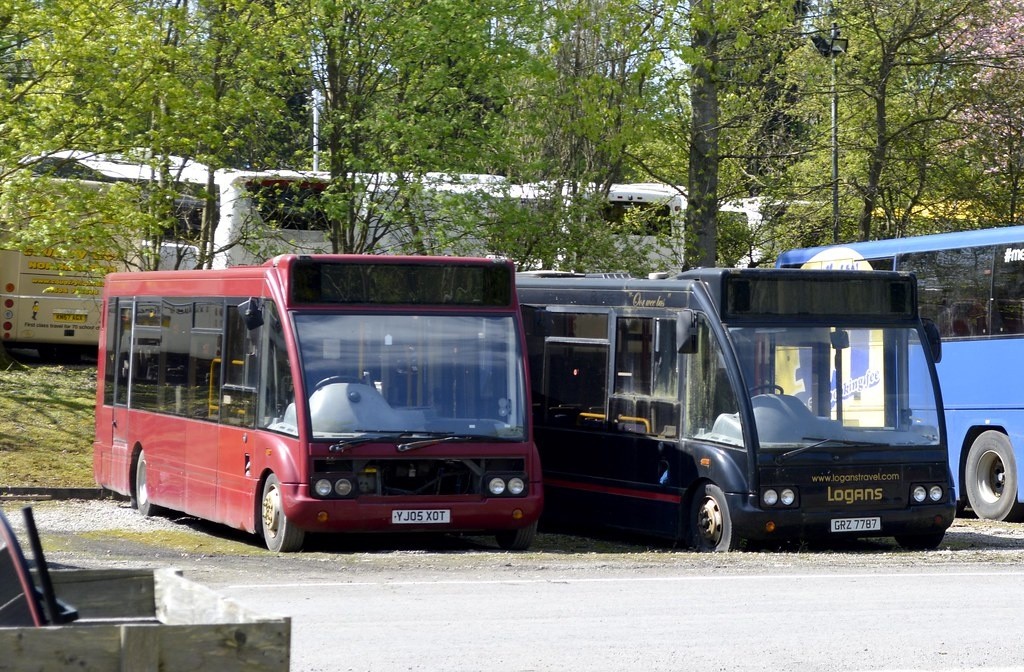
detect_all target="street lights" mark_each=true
[810,20,849,242]
[310,87,326,171]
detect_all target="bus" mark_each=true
[204,164,835,271]
[513,261,958,554]
[771,223,1023,523]
[90,251,548,557]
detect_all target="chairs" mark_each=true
[531,397,680,437]
[920,299,1024,338]
